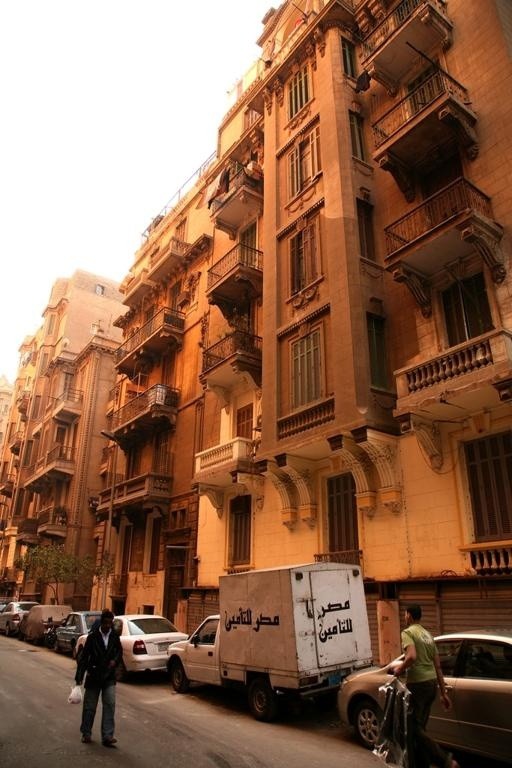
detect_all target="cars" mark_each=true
[75,614,189,681]
[18,605,72,646]
[0,602,39,638]
[338,628,512,762]
[52,611,103,653]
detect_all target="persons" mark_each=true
[74,610,123,744]
[394,603,460,768]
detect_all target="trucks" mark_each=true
[168,562,373,722]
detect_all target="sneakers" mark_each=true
[102,736,116,744]
[81,734,91,742]
